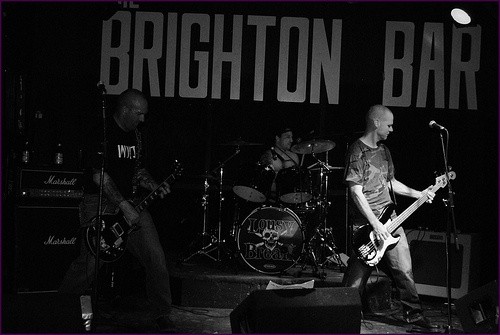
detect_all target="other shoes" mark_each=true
[404,308,424,322]
[152,316,176,329]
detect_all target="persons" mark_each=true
[342,105,440,329]
[265,127,299,171]
[80,89,175,328]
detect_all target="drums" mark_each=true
[233,159,277,203]
[236,205,306,275]
[277,166,314,203]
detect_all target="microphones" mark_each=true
[429,120,446,131]
[270,147,278,160]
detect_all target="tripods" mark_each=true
[187,149,245,274]
[296,154,348,280]
[406,131,466,335]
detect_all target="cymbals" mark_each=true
[292,140,336,155]
[312,166,345,171]
[217,140,265,146]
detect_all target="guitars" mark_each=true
[83,158,185,263]
[351,165,456,269]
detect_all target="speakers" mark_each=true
[14,205,84,294]
[229,286,360,334]
[454,280,498,332]
